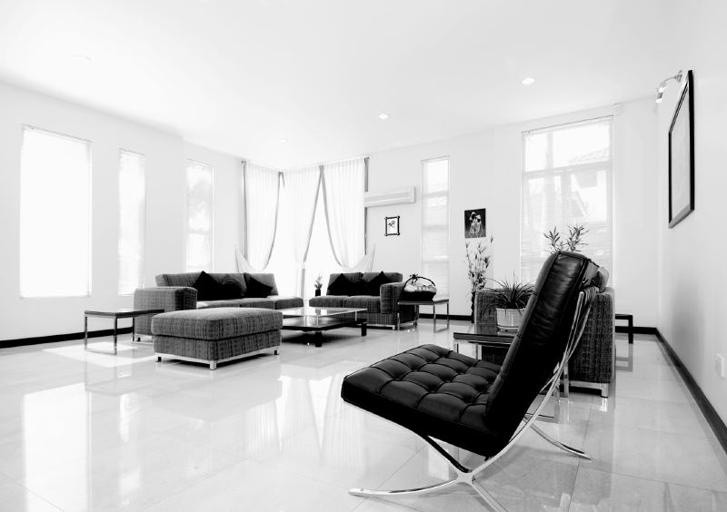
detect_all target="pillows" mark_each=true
[327,271,392,296]
[190,270,222,301]
[221,273,246,299]
[247,276,273,299]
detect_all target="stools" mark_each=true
[474,266,616,400]
[150,307,284,369]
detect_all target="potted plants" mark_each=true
[478,274,536,331]
[464,231,495,323]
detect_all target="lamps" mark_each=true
[656,69,683,104]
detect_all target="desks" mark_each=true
[83,308,165,355]
[396,299,450,333]
[452,322,573,425]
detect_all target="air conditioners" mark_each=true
[361,186,417,211]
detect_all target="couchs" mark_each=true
[132,272,304,342]
[308,271,419,330]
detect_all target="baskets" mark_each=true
[402,275,437,301]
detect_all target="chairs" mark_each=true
[339,250,601,512]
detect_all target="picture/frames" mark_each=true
[384,215,401,236]
[667,69,696,228]
